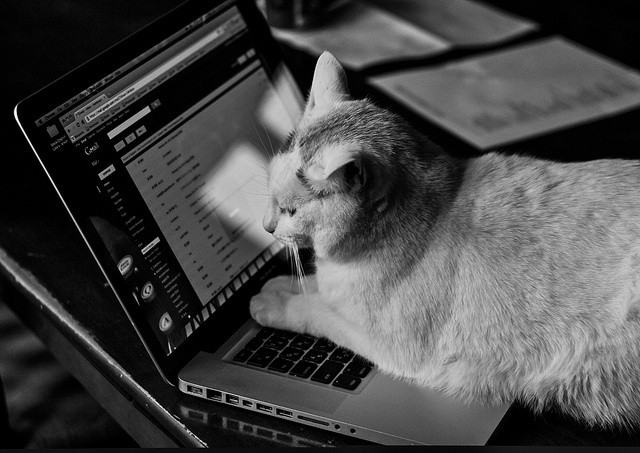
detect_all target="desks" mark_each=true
[0,0,639,452]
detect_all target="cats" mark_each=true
[249,50,640,429]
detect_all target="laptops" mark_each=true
[14,0,518,447]
